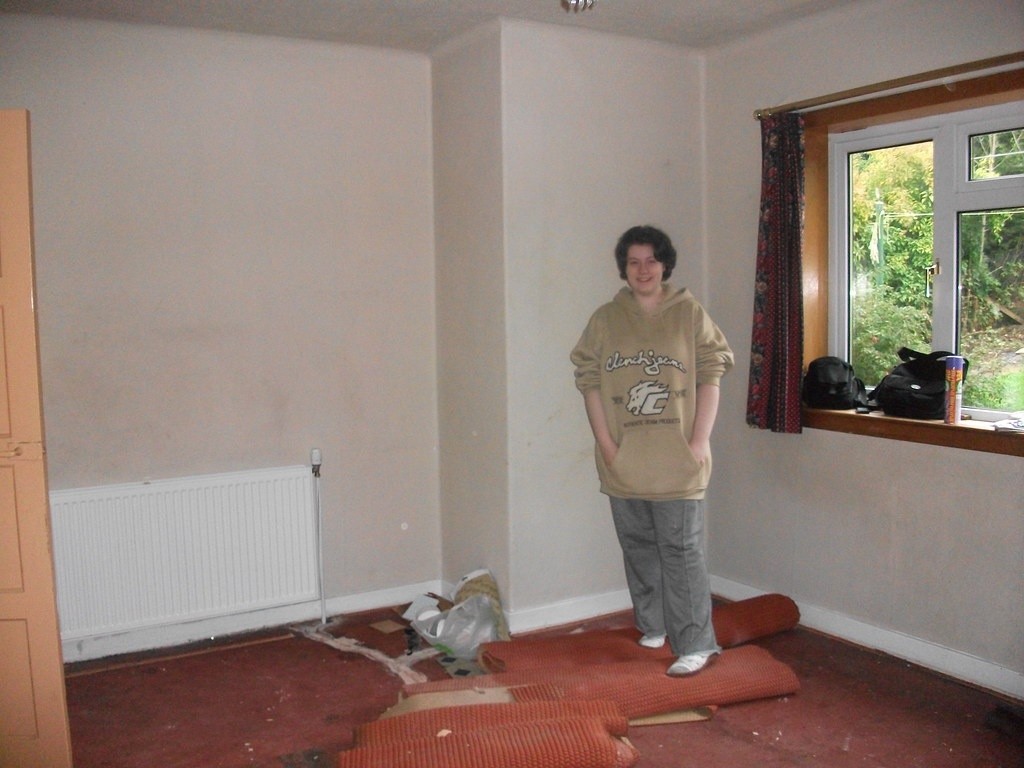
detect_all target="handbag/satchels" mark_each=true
[873,347,969,419]
[802,355,867,410]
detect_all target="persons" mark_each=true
[570,227,735,675]
